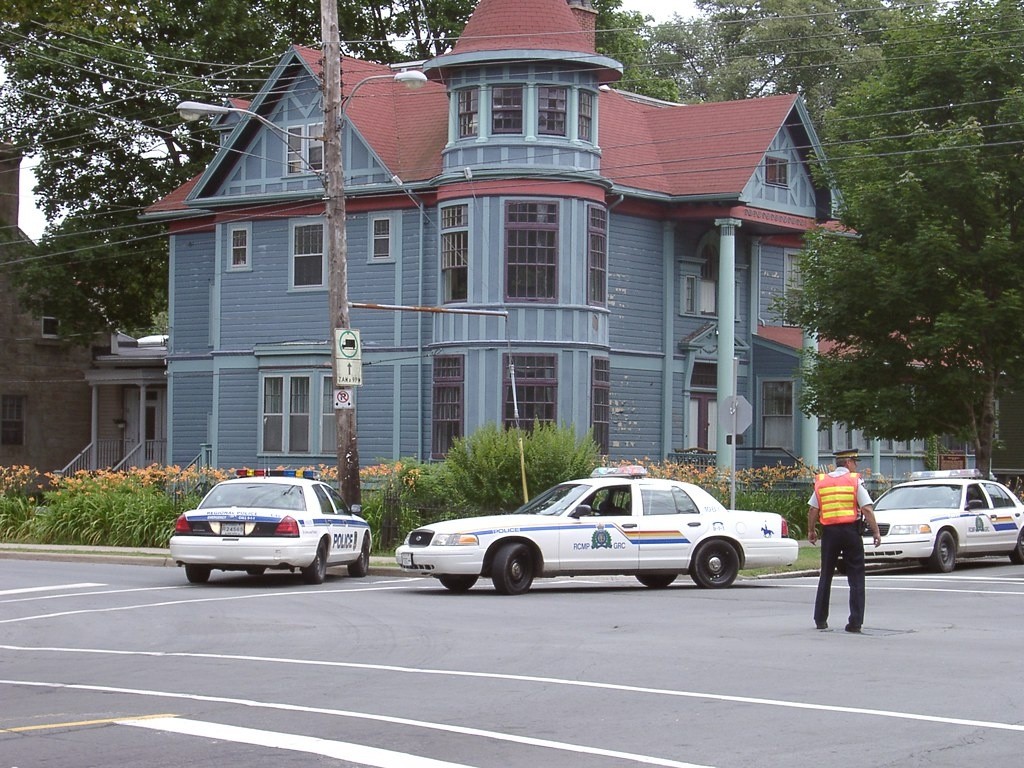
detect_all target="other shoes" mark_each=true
[845,624,860,632]
[817,622,828,629]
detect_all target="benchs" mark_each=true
[675,448,717,468]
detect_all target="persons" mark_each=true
[807,448,881,634]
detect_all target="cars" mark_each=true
[169,468,372,584]
[837,469,1024,573]
[394,466,800,597]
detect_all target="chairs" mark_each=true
[599,502,616,516]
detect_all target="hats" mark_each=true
[833,449,862,462]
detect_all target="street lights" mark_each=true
[321,70,428,515]
[177,102,363,515]
